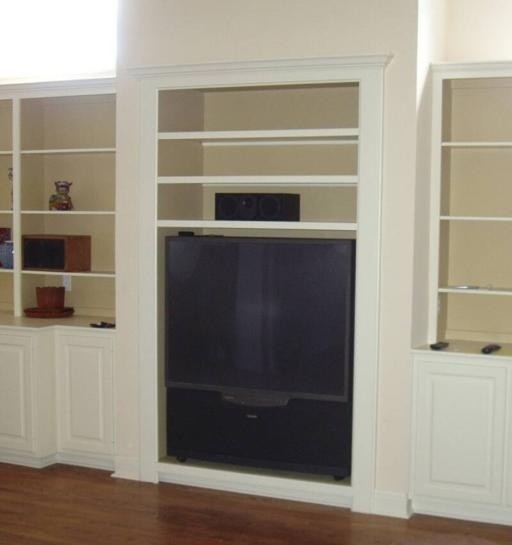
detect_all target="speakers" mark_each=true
[215,193,300,220]
[21,234,91,272]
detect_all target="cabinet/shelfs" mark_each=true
[411,59,511,527]
[1,75,117,470]
[122,55,393,514]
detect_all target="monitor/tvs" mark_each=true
[165,237,354,403]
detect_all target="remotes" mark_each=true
[482,344,500,352]
[431,342,448,350]
